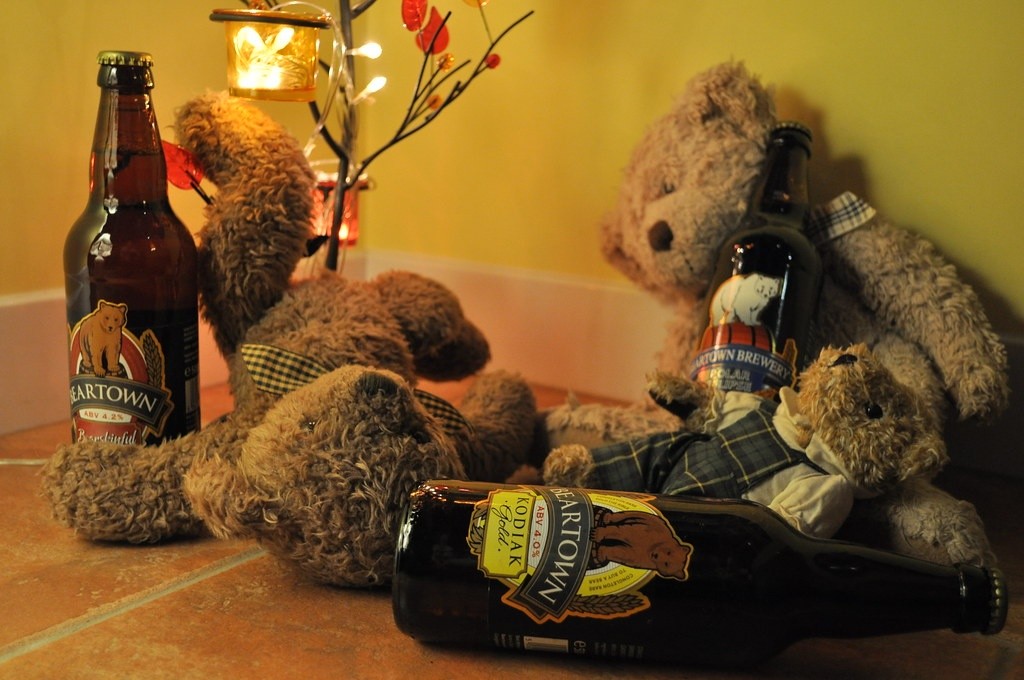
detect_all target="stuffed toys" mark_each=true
[541,325,946,538]
[34,91,542,588]
[597,59,1022,570]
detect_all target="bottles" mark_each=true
[391,475,1010,666]
[692,119,828,399]
[64,50,201,452]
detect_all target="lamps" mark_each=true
[212,0,384,157]
